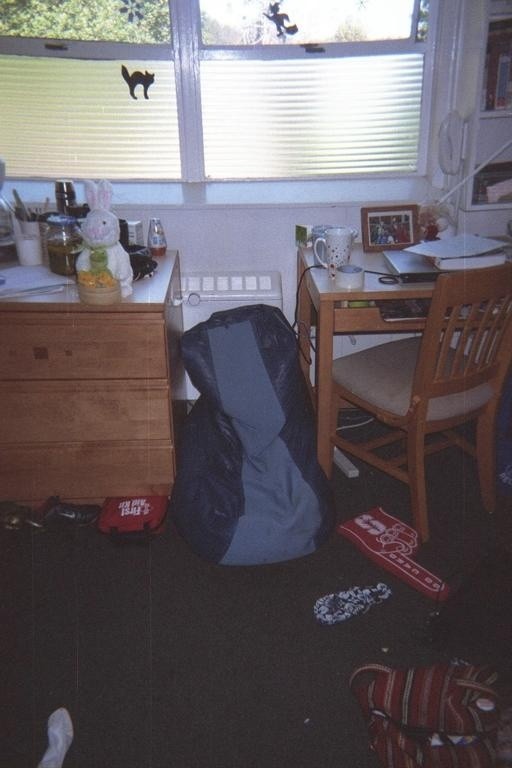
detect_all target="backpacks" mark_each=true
[169,303,336,567]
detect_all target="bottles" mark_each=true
[55,178,75,212]
[147,217,167,256]
[46,216,85,276]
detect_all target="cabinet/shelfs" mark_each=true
[1,248,187,509]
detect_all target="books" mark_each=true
[426,249,506,270]
[378,299,473,323]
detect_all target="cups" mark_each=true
[313,227,352,279]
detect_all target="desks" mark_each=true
[295,244,511,481]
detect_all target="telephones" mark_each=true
[439,112,462,175]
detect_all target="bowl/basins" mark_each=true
[78,279,122,306]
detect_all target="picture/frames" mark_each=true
[361,204,418,252]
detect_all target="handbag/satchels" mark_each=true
[97,493,169,536]
[339,663,512,767]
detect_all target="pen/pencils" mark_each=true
[9,188,49,222]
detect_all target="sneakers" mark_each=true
[37,499,101,528]
[0,509,49,539]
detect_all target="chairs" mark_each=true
[332,262,512,546]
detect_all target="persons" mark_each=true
[374,217,410,244]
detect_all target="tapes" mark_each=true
[336,264,365,289]
